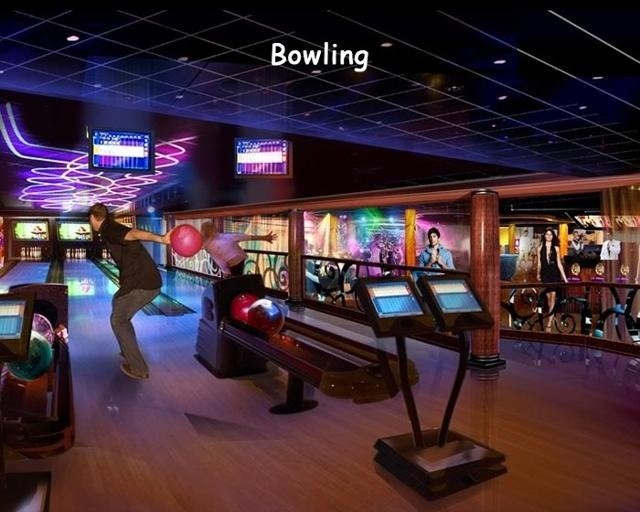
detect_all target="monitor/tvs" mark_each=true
[0,291,38,362]
[354,276,437,337]
[12,221,49,241]
[89,127,155,174]
[416,274,494,332]
[234,138,293,179]
[57,221,93,242]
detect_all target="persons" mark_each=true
[599,231,621,261]
[305,231,405,278]
[200,222,277,279]
[418,227,455,275]
[517,229,531,272]
[572,230,584,256]
[535,228,568,332]
[88,202,174,381]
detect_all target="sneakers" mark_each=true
[120,363,150,379]
